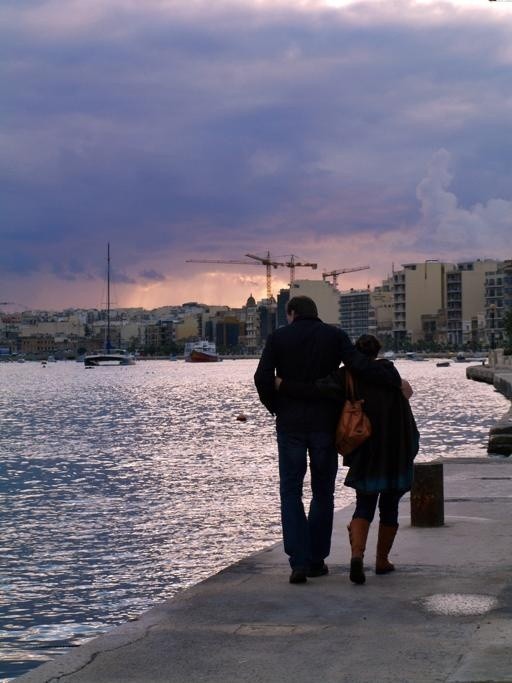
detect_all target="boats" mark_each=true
[185,338,219,362]
[383,350,472,367]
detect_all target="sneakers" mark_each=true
[289,562,328,584]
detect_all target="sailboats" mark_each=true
[83,242,136,366]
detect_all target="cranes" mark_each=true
[184,250,317,334]
[321,265,370,288]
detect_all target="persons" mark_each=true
[337,334,421,585]
[253,297,376,583]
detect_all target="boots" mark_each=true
[347,519,370,585]
[376,523,399,575]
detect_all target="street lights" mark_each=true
[489,303,497,368]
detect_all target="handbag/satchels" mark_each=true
[334,399,372,457]
